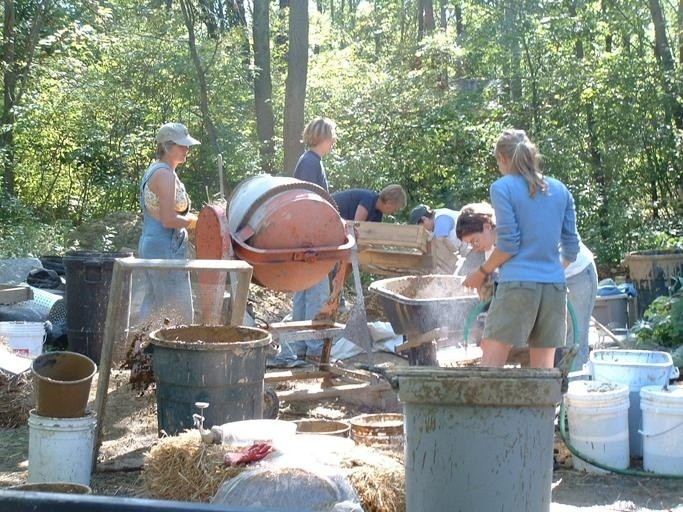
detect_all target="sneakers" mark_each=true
[304,351,345,369]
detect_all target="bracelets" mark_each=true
[475,265,492,279]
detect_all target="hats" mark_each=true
[408,202,429,226]
[154,121,201,147]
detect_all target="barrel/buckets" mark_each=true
[350,413,403,446]
[620,250,683,328]
[438,347,483,368]
[563,380,630,475]
[31,351,98,417]
[591,293,630,334]
[63,251,134,365]
[2,482,92,496]
[28,410,98,486]
[389,364,562,512]
[148,323,273,439]
[293,419,349,439]
[583,348,680,457]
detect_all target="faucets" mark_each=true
[639,385,683,475]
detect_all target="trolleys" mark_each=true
[364,270,502,366]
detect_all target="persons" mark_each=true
[133,121,199,337]
[327,184,407,298]
[289,115,337,364]
[408,204,487,277]
[454,199,600,371]
[458,126,579,369]
